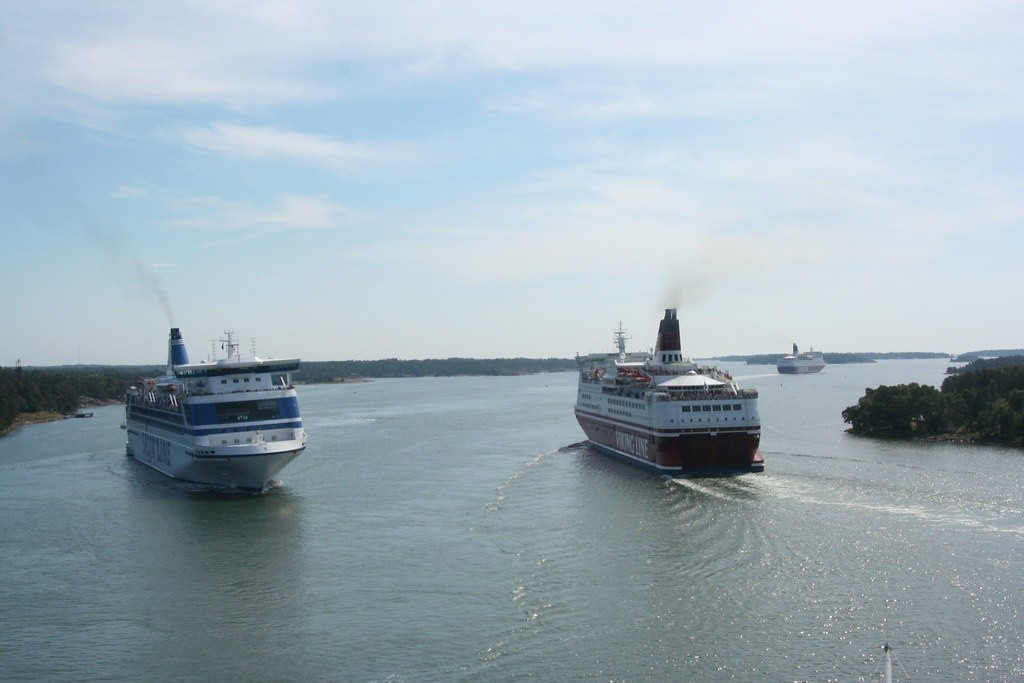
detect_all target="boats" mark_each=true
[573,306,766,476]
[122,328,308,493]
[776,343,826,374]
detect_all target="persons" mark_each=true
[910,418,917,431]
